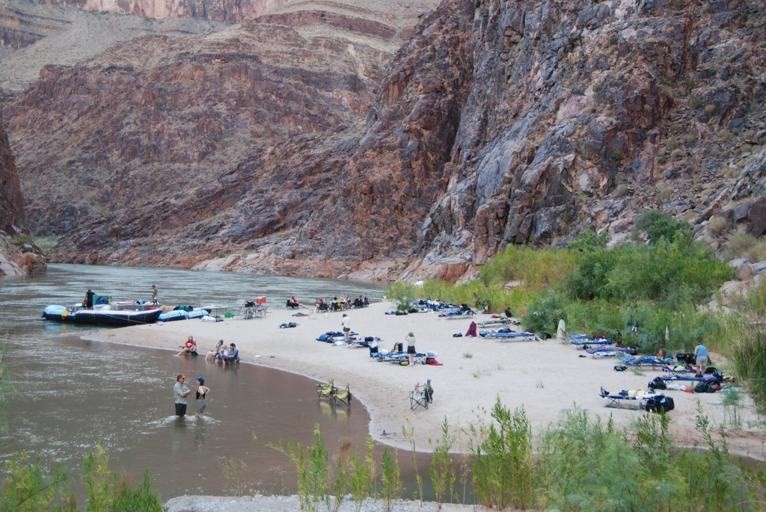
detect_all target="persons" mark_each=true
[404,331,416,368]
[173,373,191,418]
[194,376,209,418]
[150,285,158,306]
[176,335,197,357]
[692,341,709,377]
[314,292,370,313]
[290,295,299,309]
[341,313,350,341]
[223,343,240,364]
[214,344,227,365]
[84,288,95,310]
[204,339,223,361]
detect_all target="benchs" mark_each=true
[566,333,724,416]
[370,325,537,365]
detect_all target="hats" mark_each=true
[197,377,204,382]
[177,374,183,381]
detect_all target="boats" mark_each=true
[42,300,211,327]
